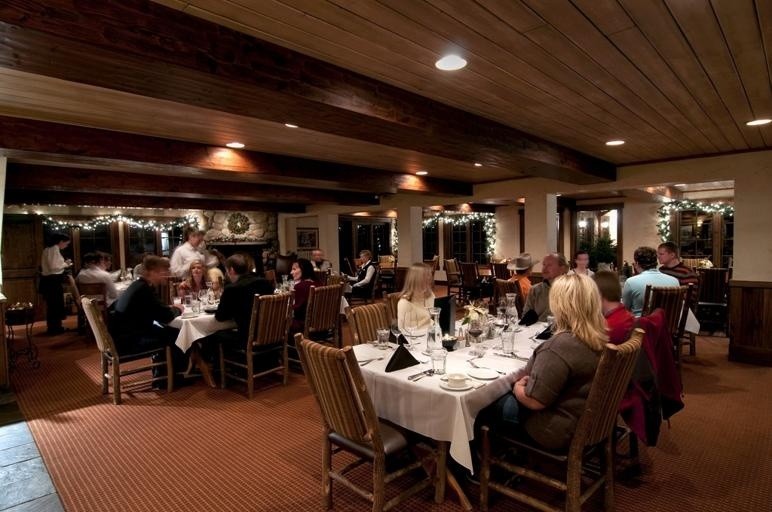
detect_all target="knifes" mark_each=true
[357,357,385,366]
[408,369,433,381]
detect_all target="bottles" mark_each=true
[427,307,443,353]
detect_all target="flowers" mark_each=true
[461,298,489,320]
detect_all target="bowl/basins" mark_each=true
[441,337,457,349]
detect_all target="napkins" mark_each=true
[389,323,410,345]
[518,308,539,327]
[533,323,555,341]
[385,344,420,373]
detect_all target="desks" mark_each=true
[5,304,42,370]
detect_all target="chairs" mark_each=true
[64,252,733,358]
[474,326,645,510]
[639,280,694,393]
[79,294,174,404]
[292,332,450,510]
[620,306,670,444]
[286,280,345,373]
[218,289,292,399]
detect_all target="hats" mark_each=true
[505,252,542,272]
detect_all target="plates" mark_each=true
[469,368,498,380]
[440,383,475,391]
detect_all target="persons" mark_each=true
[465,274,613,487]
[133,227,332,390]
[40,234,73,335]
[114,254,196,389]
[591,242,699,345]
[76,251,119,310]
[397,262,436,344]
[344,250,377,299]
[506,251,618,323]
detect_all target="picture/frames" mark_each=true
[295,226,321,251]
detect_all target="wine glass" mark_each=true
[275,275,293,294]
[457,292,519,358]
[547,314,558,334]
[391,311,418,350]
[173,281,220,319]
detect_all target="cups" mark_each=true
[376,328,390,350]
[447,374,470,387]
[430,346,447,375]
